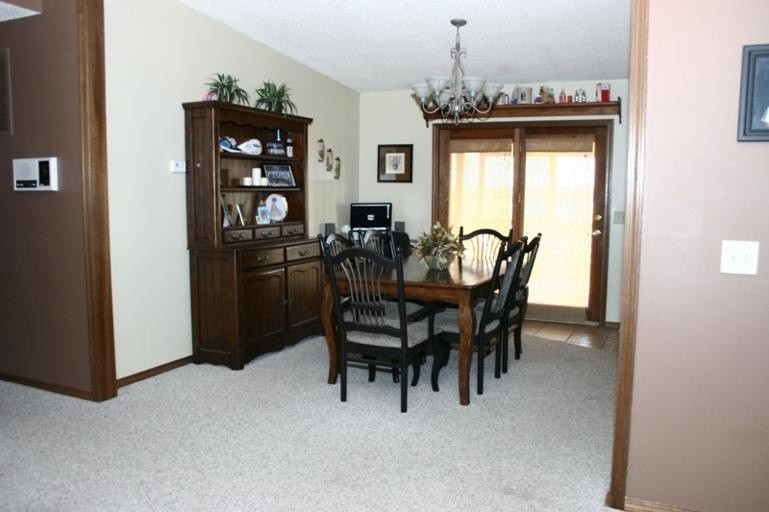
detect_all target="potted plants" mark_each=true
[254,82,298,119]
[203,73,250,106]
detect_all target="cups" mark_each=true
[512,87,532,105]
[243,167,267,186]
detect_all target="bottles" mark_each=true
[537,82,610,104]
[274,129,283,143]
[286,132,294,157]
[256,200,264,217]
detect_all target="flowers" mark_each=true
[416,222,466,270]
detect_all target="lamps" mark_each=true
[410,19,504,127]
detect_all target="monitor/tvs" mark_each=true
[350,203,392,232]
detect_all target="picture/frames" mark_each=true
[378,144,413,182]
[0,47,14,137]
[737,43,769,143]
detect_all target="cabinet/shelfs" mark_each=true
[190,239,325,370]
[182,100,313,249]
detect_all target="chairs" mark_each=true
[425,227,542,394]
[358,228,397,262]
[321,244,447,413]
[317,231,425,383]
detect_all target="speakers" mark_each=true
[395,221,405,232]
[324,223,334,237]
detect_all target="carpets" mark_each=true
[0,333,620,511]
